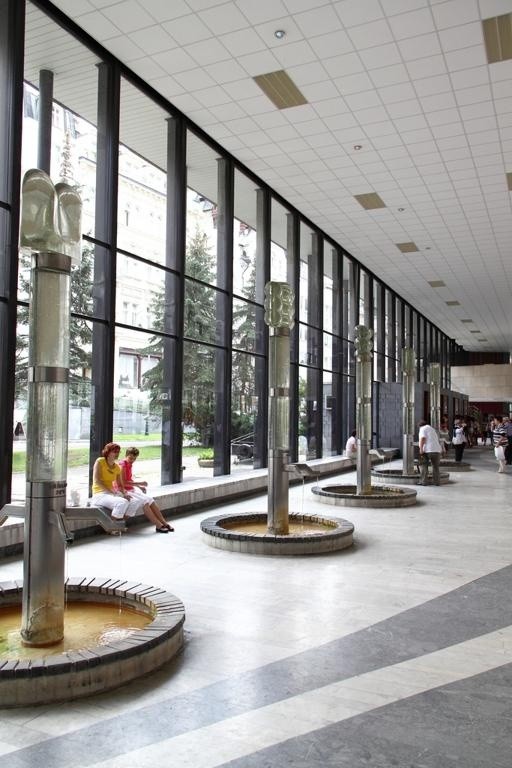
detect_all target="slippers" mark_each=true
[166,524,174,531]
[156,526,169,533]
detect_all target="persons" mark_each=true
[342,414,512,486]
[92,442,138,536]
[12,422,25,436]
[111,447,174,532]
